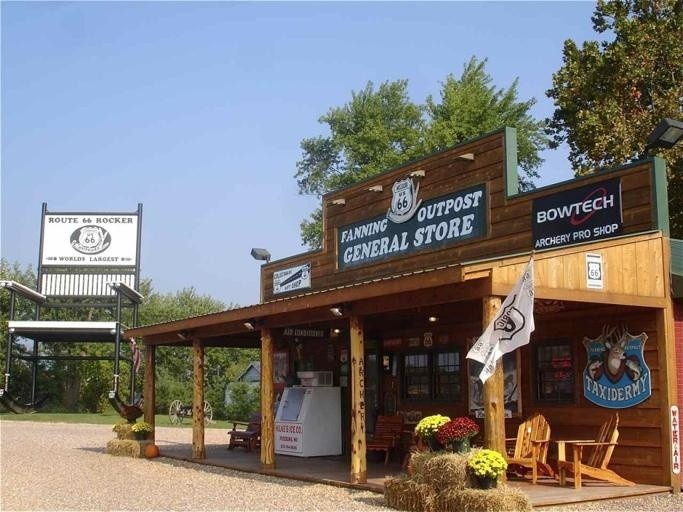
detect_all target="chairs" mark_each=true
[505,407,637,495]
[367,413,404,467]
[225,407,262,452]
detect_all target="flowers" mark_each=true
[414,413,509,482]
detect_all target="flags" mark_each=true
[465,258,537,385]
[129,338,140,374]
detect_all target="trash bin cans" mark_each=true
[364,406,383,464]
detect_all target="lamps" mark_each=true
[243,318,257,331]
[176,329,188,341]
[250,247,273,265]
[329,303,345,316]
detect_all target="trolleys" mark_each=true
[168,400,216,426]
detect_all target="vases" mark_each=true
[477,478,498,489]
